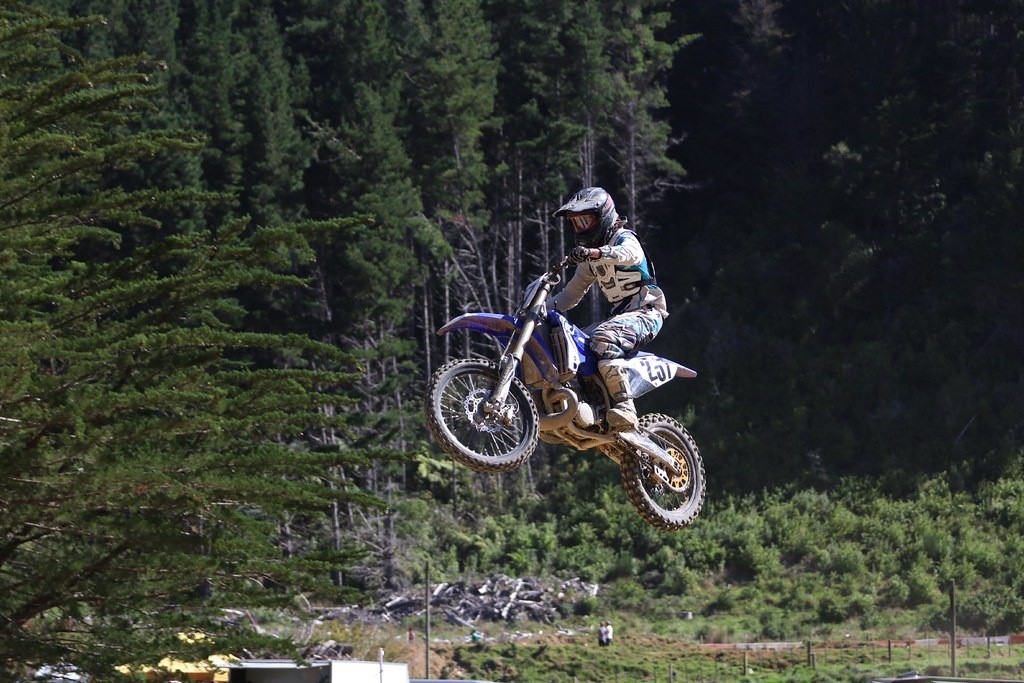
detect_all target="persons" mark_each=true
[598,621,606,646]
[408,627,415,642]
[547,187,665,432]
[604,621,612,645]
[472,630,481,643]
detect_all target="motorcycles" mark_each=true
[424,245,709,532]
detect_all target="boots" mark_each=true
[598,358,639,428]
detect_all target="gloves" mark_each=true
[568,246,590,265]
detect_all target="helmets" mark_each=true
[553,187,619,246]
